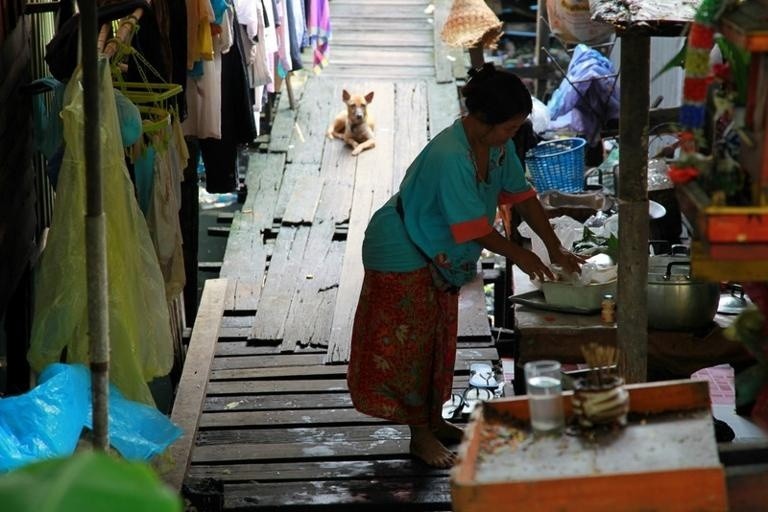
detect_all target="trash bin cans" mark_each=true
[525,137,588,195]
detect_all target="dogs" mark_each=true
[327,89,377,156]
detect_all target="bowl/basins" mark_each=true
[647,199,667,223]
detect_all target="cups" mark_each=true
[525,359,566,443]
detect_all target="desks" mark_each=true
[505,237,712,398]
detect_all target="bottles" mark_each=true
[600,294,617,324]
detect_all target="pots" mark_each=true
[646,241,721,331]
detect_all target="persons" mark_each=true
[344,59,590,471]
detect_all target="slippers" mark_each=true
[440,393,466,420]
[469,363,501,389]
[462,387,496,417]
